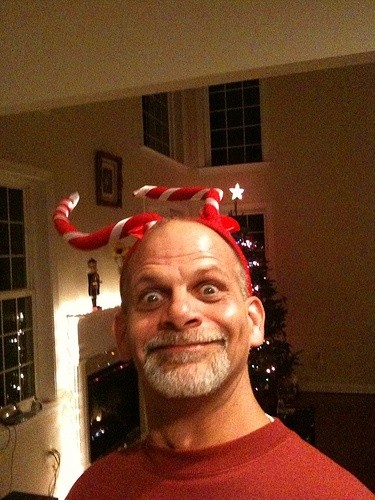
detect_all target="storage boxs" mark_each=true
[276,395,316,444]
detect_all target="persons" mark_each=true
[54,186,375,500]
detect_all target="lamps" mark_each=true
[230,183,244,216]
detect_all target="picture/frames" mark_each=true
[96,151,123,208]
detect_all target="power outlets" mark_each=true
[47,445,57,461]
